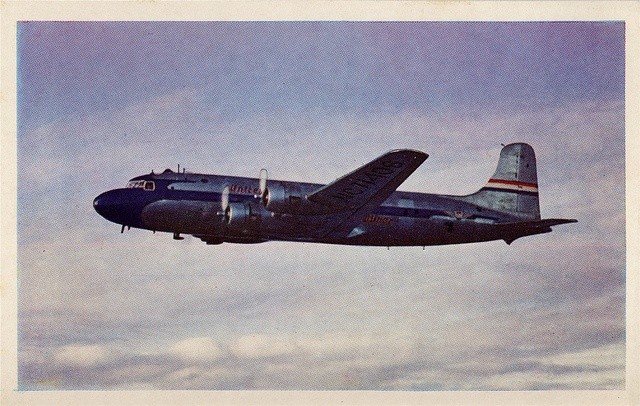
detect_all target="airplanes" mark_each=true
[93,142,579,250]
